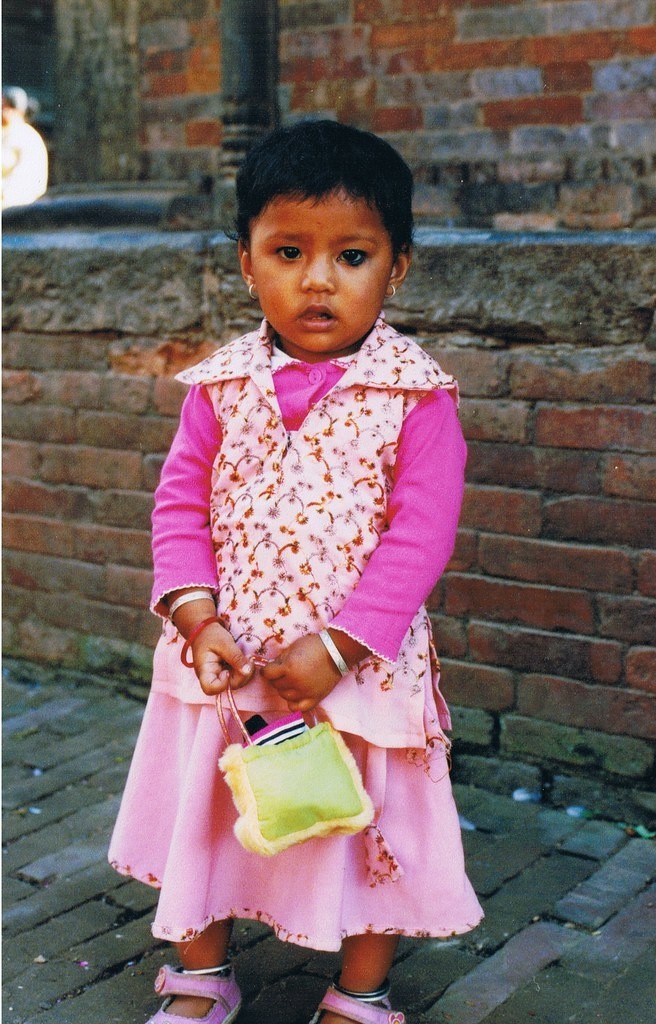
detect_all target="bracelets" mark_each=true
[180,616,225,668]
[318,628,350,678]
[168,591,214,621]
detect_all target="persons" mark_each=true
[108,119,484,1024]
[2,86,48,209]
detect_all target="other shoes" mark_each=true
[308,986,407,1024]
[144,964,242,1024]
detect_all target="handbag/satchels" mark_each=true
[216,657,374,856]
